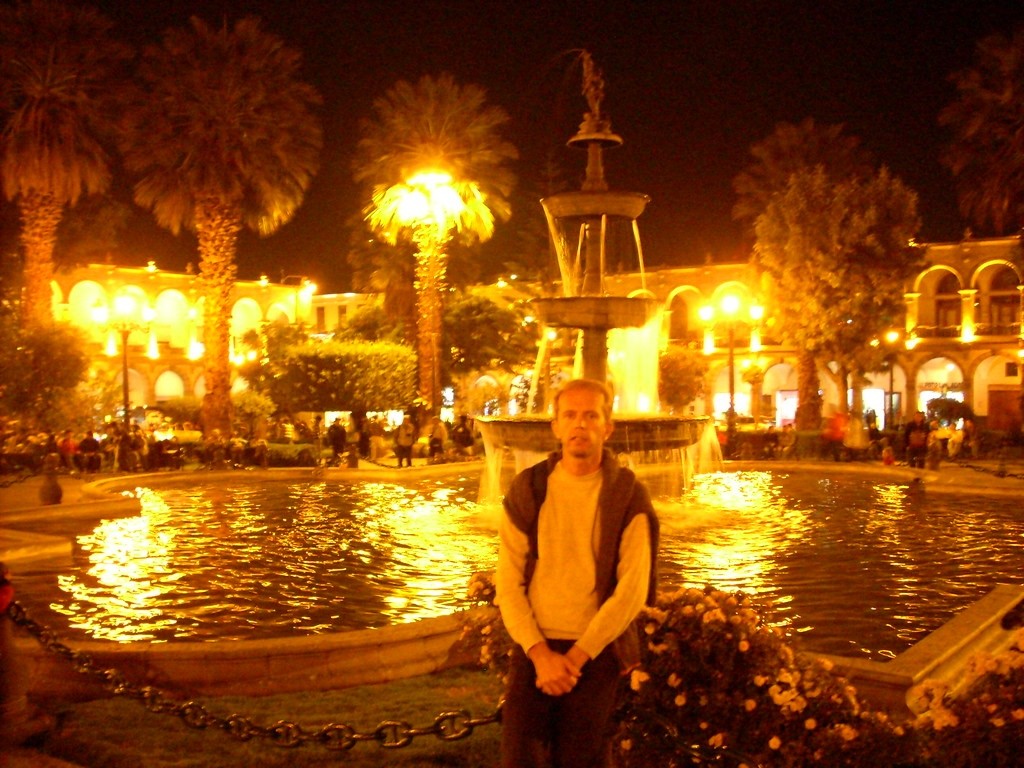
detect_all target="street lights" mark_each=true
[91,299,160,471]
[867,329,918,430]
[389,170,463,448]
[695,293,766,460]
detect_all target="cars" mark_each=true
[718,414,775,435]
[144,411,173,425]
[154,421,204,445]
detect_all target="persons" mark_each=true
[0,562,56,756]
[497,379,660,768]
[1,405,1024,477]
